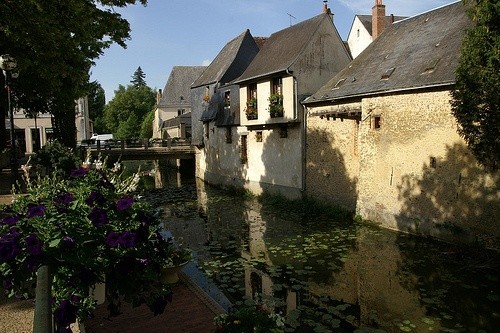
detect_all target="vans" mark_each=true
[89,133,115,150]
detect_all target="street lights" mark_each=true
[0,53,20,194]
